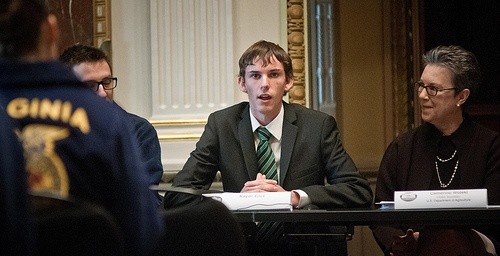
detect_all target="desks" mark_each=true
[223,205,500,225]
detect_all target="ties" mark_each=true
[255,127,285,256]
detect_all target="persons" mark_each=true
[370,45,500,256]
[0,0,165,256]
[165,40,374,231]
[59,44,163,188]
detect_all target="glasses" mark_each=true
[81,77,117,92]
[415,80,457,97]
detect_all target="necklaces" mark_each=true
[436,150,457,162]
[433,162,460,187]
